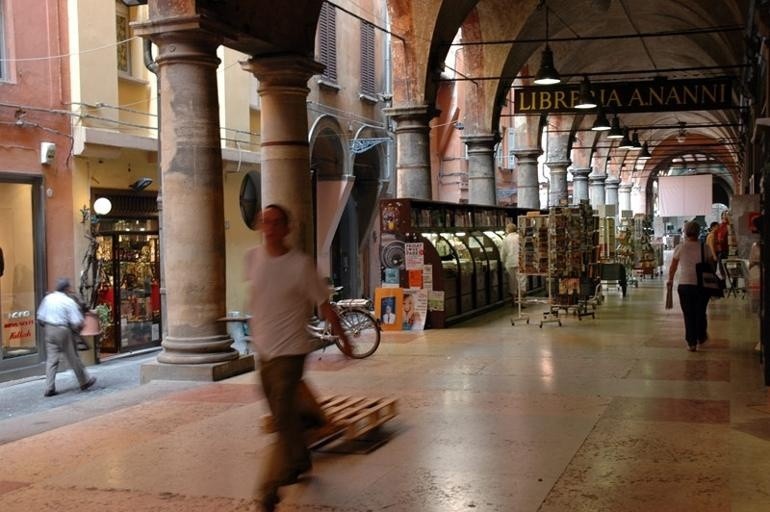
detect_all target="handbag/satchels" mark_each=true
[695,260,724,298]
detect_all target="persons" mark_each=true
[705,222,720,274]
[401,294,422,330]
[498,222,531,310]
[716,209,730,289]
[238,202,355,511]
[119,271,135,301]
[129,257,155,323]
[33,275,98,398]
[666,221,717,352]
[382,306,395,324]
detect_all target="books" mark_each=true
[411,208,513,227]
[516,203,600,279]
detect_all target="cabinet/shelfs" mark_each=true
[511,207,668,328]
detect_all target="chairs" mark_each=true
[723,259,752,299]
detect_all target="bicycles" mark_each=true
[305,285,383,359]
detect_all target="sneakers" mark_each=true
[44,390,58,396]
[79,377,96,390]
[248,483,278,512]
[686,344,697,351]
[276,458,313,485]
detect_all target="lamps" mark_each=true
[534,1,651,160]
